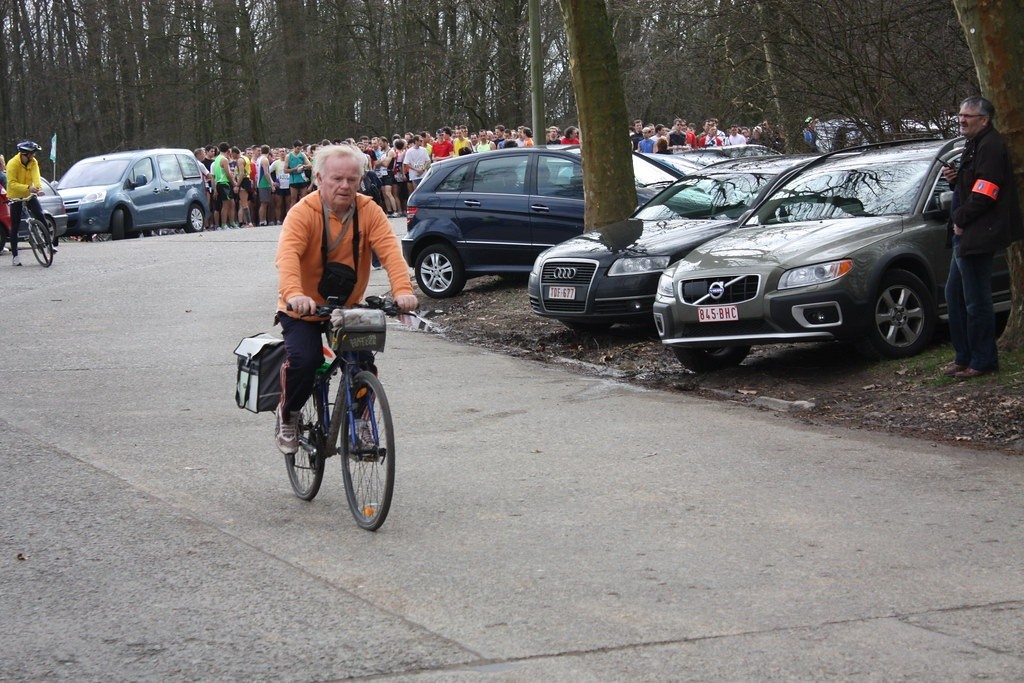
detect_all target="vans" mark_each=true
[54,148,211,240]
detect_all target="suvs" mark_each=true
[400,144,784,299]
[654,135,1011,373]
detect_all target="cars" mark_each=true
[528,150,871,333]
[0,175,68,252]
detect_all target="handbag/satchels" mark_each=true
[392,168,398,175]
[317,262,356,306]
[235,333,286,413]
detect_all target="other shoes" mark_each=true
[374,265,382,270]
[1,247,9,253]
[203,219,282,232]
[943,364,963,376]
[387,210,407,217]
[954,364,991,379]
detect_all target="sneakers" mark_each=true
[347,420,377,459]
[274,400,300,455]
[12,256,23,266]
[47,245,58,253]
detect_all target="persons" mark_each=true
[943,95,1023,378]
[273,144,418,462]
[0,155,9,255]
[5,141,58,266]
[194,117,816,231]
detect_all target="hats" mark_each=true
[436,129,444,135]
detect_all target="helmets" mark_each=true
[16,141,42,154]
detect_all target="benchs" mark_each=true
[473,169,521,195]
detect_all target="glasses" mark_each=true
[957,113,987,119]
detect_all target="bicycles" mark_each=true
[274,295,421,531]
[2,193,53,268]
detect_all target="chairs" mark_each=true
[538,164,555,195]
